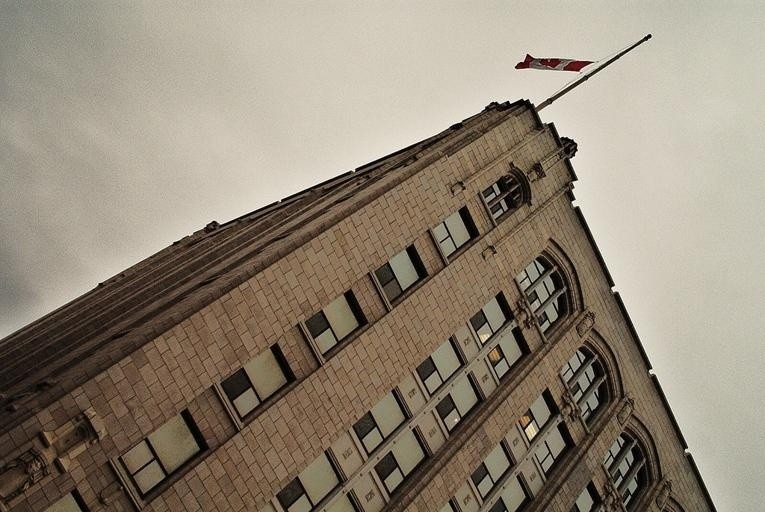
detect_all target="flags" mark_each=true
[515,53,597,74]
